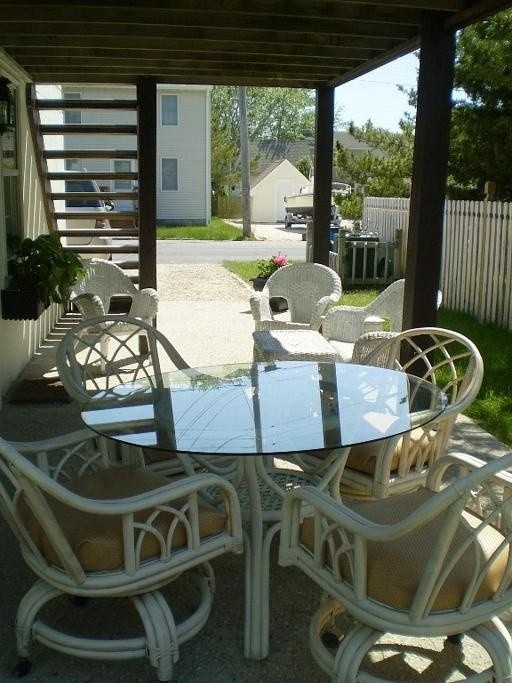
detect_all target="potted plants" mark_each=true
[1,229,89,322]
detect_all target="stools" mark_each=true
[253,328,343,362]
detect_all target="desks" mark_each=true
[81,360,449,660]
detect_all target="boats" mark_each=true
[284,193,314,216]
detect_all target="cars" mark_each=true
[64,171,115,261]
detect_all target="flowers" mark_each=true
[257,251,289,277]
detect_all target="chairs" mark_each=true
[323,279,443,368]
[304,326,485,501]
[56,315,192,422]
[0,428,246,683]
[278,451,512,683]
[250,261,341,331]
[63,259,160,374]
